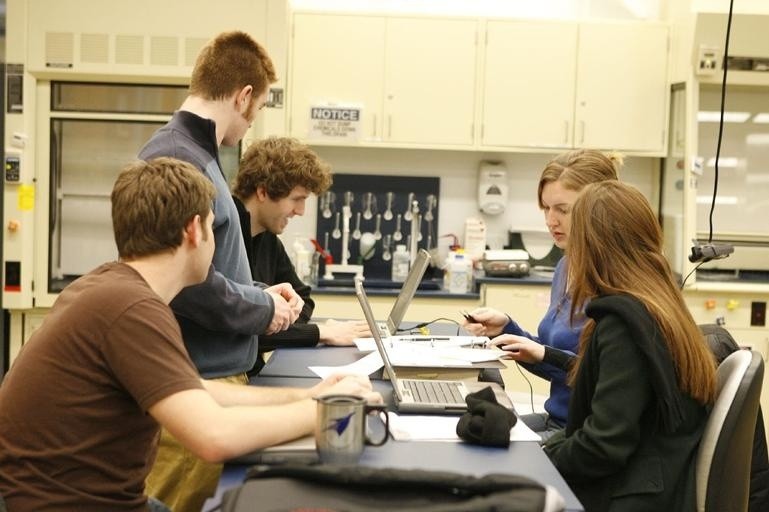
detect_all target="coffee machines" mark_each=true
[460,310,476,323]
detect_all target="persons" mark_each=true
[543,181,718,512]
[1,157,384,512]
[229,136,371,352]
[462,148,618,447]
[136,31,305,512]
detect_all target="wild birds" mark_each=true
[307,248,432,341]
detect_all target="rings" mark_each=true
[400,338,449,341]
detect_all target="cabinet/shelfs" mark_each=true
[391,244,410,282]
[442,244,471,294]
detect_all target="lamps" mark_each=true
[692,347,766,512]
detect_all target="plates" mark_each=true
[24,309,56,350]
[257,286,481,367]
[286,11,481,159]
[477,277,554,418]
[479,17,673,160]
[23,1,295,92]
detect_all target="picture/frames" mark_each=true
[199,375,590,512]
[255,315,506,392]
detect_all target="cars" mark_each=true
[295,250,322,288]
[308,392,388,465]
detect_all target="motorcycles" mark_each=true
[353,277,470,415]
